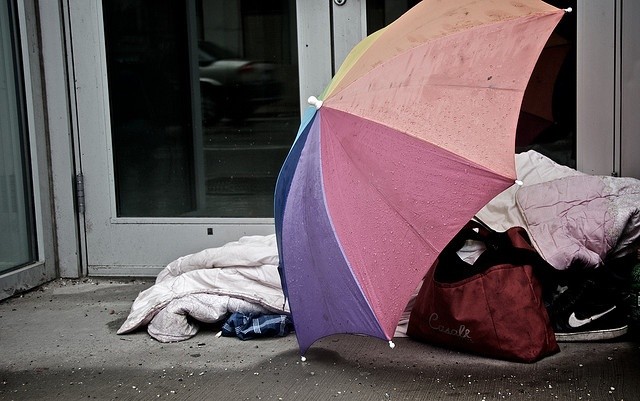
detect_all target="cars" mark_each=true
[198,39,288,128]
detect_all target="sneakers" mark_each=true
[551,305,630,342]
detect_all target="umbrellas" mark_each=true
[273,0,573,361]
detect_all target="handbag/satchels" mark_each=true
[405,218,560,365]
